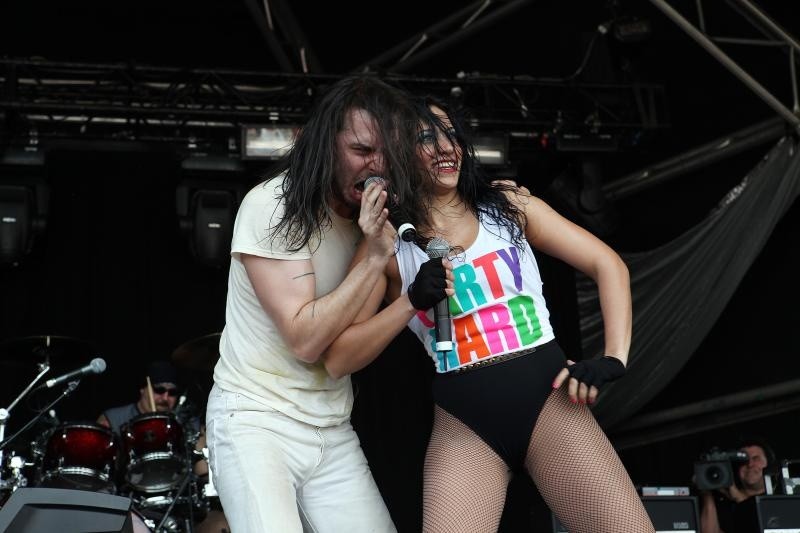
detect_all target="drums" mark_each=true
[115,411,192,493]
[33,421,119,491]
[137,487,212,533]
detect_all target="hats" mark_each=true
[143,362,179,387]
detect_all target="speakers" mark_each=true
[0,485,134,533]
[736,494,800,533]
[552,496,702,533]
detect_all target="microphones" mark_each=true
[425,236,455,354]
[362,174,419,243]
[44,356,107,390]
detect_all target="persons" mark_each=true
[700,435,768,533]
[318,92,656,533]
[204,79,532,533]
[100,363,209,475]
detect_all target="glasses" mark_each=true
[153,386,178,396]
